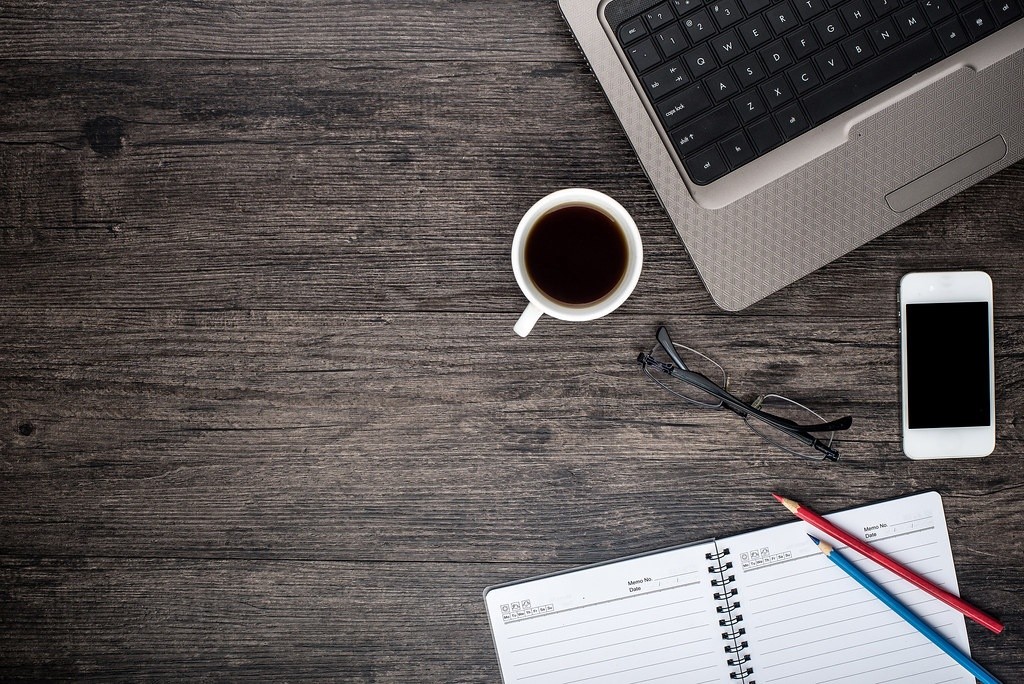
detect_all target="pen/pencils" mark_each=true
[769,490,1004,635]
[806,533,1004,684]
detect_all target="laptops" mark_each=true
[554,0,1024,311]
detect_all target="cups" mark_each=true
[511,188,643,338]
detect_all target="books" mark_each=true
[481,487,980,684]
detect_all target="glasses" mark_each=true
[637,327,851,462]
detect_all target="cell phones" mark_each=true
[895,269,996,459]
